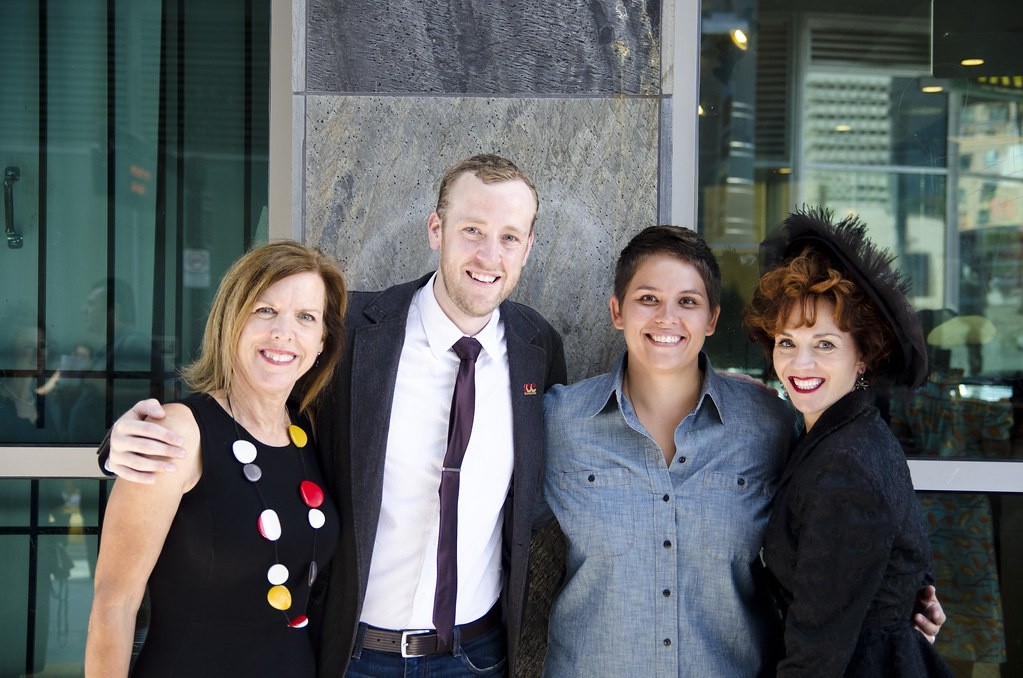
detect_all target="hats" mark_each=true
[758,203,931,392]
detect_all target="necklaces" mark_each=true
[626,368,703,423]
[228,393,327,630]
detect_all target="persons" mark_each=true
[96,153,568,678]
[81,239,348,678]
[740,219,933,678]
[887,307,1023,678]
[541,224,947,678]
[0,278,197,678]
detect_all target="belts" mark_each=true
[354,600,502,658]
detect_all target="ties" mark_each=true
[433,336,482,645]
